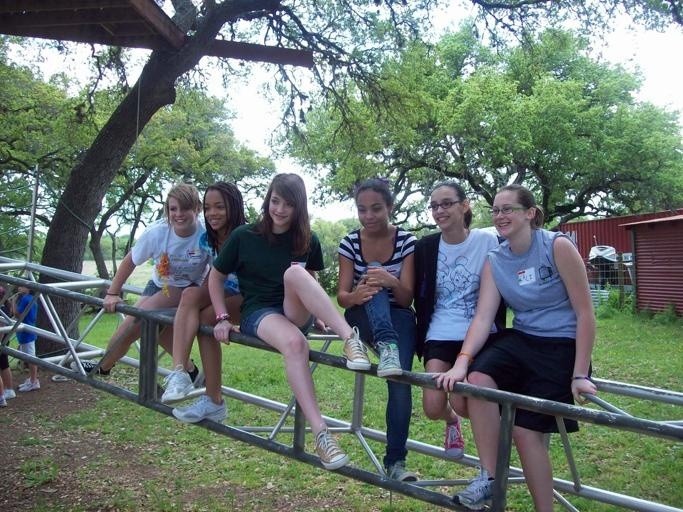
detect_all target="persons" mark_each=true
[0,286,16,408]
[12,281,41,392]
[208,173,372,469]
[338,178,419,482]
[71,185,210,394]
[415,182,507,459]
[431,184,597,512]
[162,181,244,423]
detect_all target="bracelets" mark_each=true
[214,313,230,322]
[456,352,476,361]
[105,292,120,296]
[570,376,590,380]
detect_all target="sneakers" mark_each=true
[387,461,417,482]
[70,359,115,377]
[4,389,17,400]
[172,394,227,424]
[313,423,349,470]
[160,358,206,404]
[444,420,464,461]
[18,376,40,392]
[376,341,403,378]
[452,465,495,510]
[0,395,8,407]
[341,325,372,371]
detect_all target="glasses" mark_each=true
[427,200,462,211]
[488,205,528,217]
